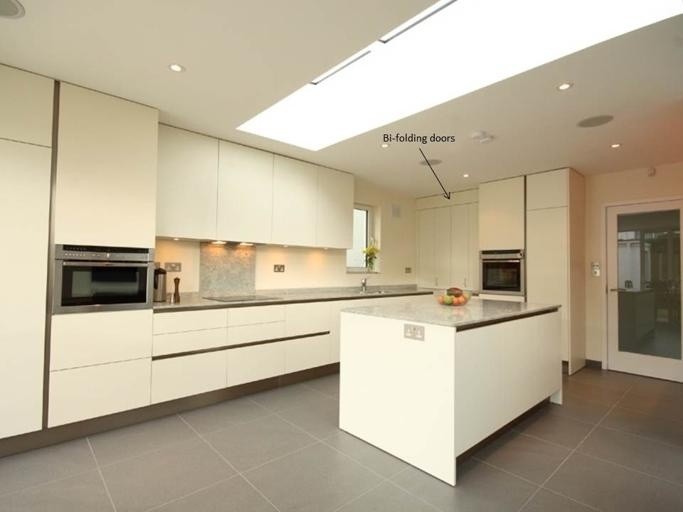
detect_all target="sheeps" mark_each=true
[361,277,367,292]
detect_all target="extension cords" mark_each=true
[153,267,167,303]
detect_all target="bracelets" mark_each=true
[434,290,472,307]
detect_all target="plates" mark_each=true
[156,122,355,249]
[415,187,479,293]
[152,301,330,405]
[526,167,586,377]
[0,62,55,441]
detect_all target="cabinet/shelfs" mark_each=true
[173,277,181,303]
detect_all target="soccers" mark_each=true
[438,294,467,305]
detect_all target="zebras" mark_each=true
[52,244,155,315]
[479,249,526,296]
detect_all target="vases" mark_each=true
[362,246,379,272]
